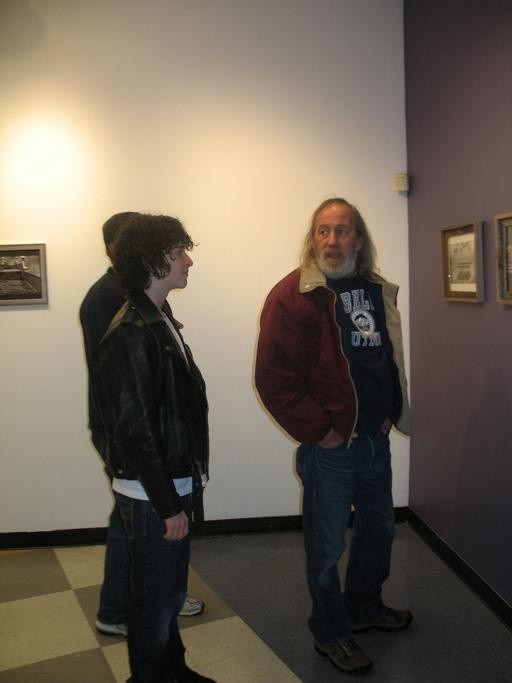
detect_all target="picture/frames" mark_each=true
[440,212,512,305]
[0,243,49,306]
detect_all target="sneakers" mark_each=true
[314,633,373,676]
[95,617,129,636]
[347,603,413,633]
[178,593,204,616]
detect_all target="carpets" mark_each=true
[189,522,511,682]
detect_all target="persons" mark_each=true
[90,212,211,681]
[79,211,206,637]
[254,197,416,675]
